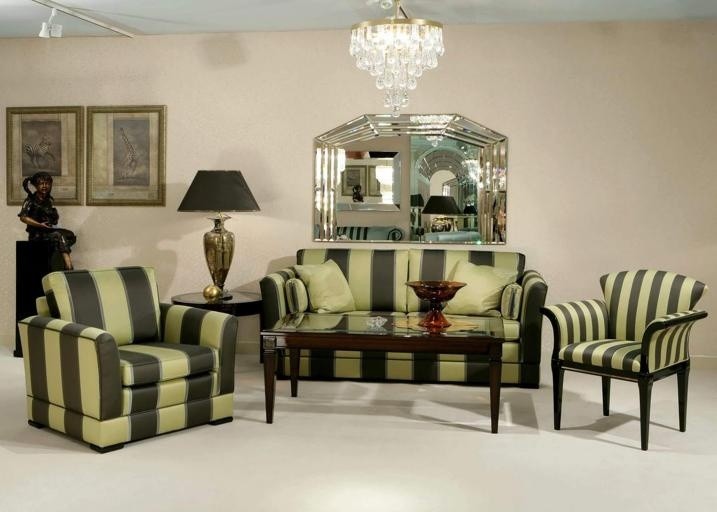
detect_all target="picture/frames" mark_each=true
[342,165,368,196]
[6,106,86,205]
[369,166,385,197]
[86,105,167,206]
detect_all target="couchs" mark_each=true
[314,225,404,241]
[259,247,548,390]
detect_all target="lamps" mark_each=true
[39,7,62,40]
[463,206,477,229]
[347,1,450,118]
[422,195,462,232]
[177,171,259,300]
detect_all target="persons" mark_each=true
[352,185,365,202]
[20,172,77,271]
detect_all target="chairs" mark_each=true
[541,269,709,449]
[18,265,238,453]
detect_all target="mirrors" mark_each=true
[312,113,509,246]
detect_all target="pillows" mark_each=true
[285,248,526,321]
[42,265,163,346]
